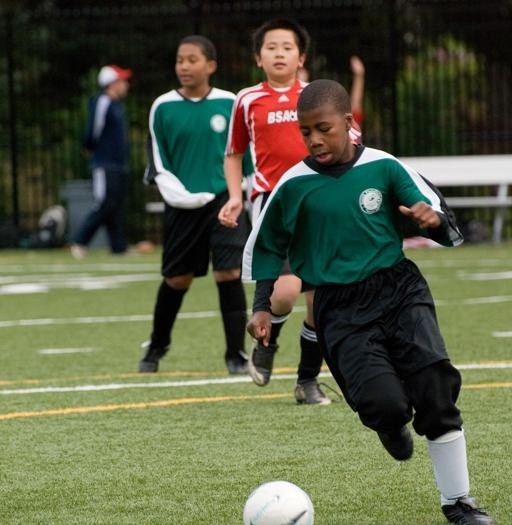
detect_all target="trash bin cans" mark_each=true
[59,178,113,249]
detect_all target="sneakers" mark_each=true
[440,493,498,525]
[225,349,249,374]
[250,338,279,387]
[294,378,332,405]
[139,346,169,373]
[379,426,413,460]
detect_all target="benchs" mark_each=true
[145,153,512,243]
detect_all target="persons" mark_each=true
[246,79,497,524]
[219,19,337,406]
[68,61,142,257]
[140,35,253,375]
[298,49,364,142]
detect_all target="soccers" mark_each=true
[244,480,313,525]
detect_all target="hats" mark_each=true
[98,64,132,88]
